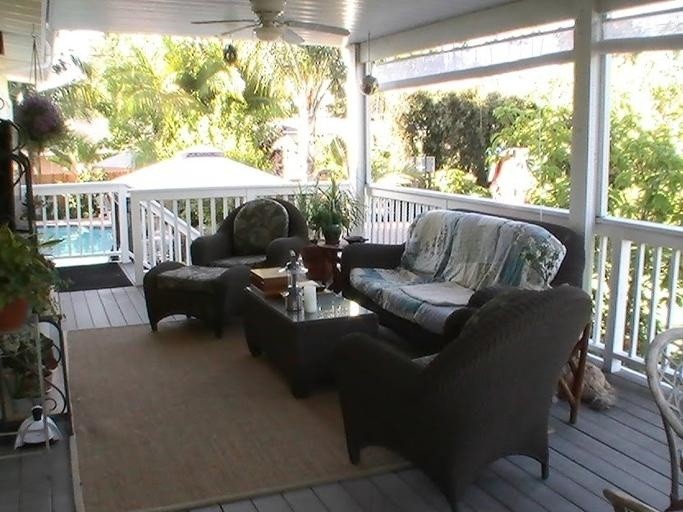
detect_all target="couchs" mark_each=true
[341,208,586,348]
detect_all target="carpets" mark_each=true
[61,321,416,511]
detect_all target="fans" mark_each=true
[191,0,351,45]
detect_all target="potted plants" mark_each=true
[1,225,71,330]
[308,178,364,246]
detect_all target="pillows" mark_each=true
[233,201,289,257]
[400,211,567,291]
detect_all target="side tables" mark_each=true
[317,238,345,286]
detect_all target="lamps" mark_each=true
[254,19,281,43]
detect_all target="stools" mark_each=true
[144,262,251,339]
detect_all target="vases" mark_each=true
[25,371,51,393]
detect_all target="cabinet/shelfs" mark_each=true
[0,289,73,452]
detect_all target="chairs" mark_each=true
[338,286,594,510]
[190,198,309,266]
[602,325,683,511]
